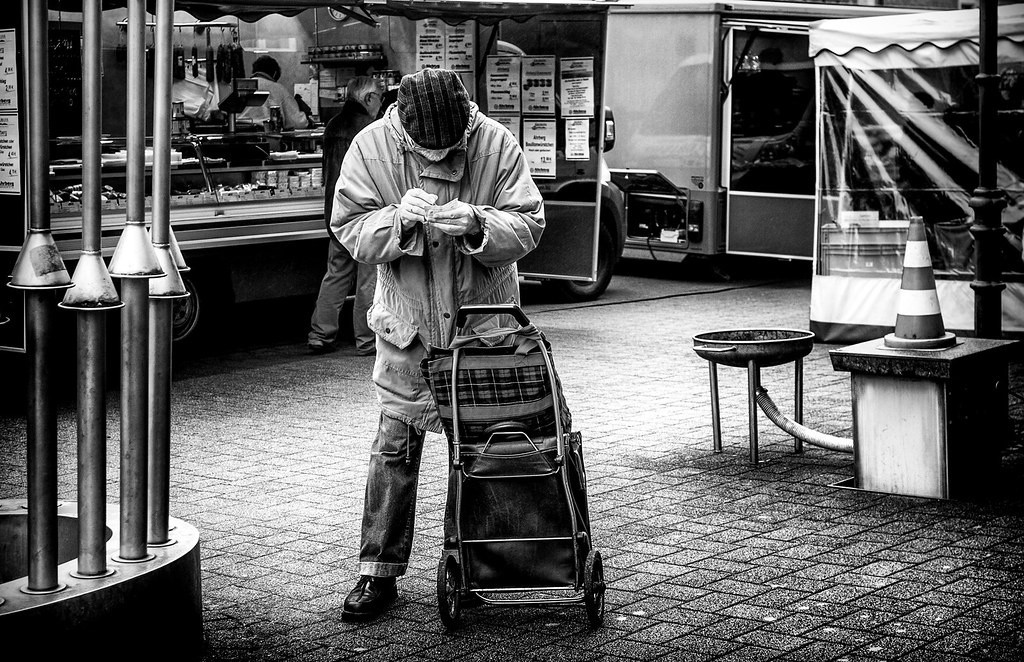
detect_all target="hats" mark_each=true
[397,67,470,150]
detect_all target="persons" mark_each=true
[236,56,312,129]
[305,76,386,357]
[330,68,548,620]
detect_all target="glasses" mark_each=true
[369,91,385,103]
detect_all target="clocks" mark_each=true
[326,6,354,23]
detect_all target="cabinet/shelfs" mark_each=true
[299,52,402,128]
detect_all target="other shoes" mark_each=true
[309,341,337,352]
[357,349,376,355]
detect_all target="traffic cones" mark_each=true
[884,216,964,352]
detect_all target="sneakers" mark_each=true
[340,576,398,618]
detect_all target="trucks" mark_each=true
[517,2,961,301]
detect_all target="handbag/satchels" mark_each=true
[441,421,591,589]
[420,325,571,440]
